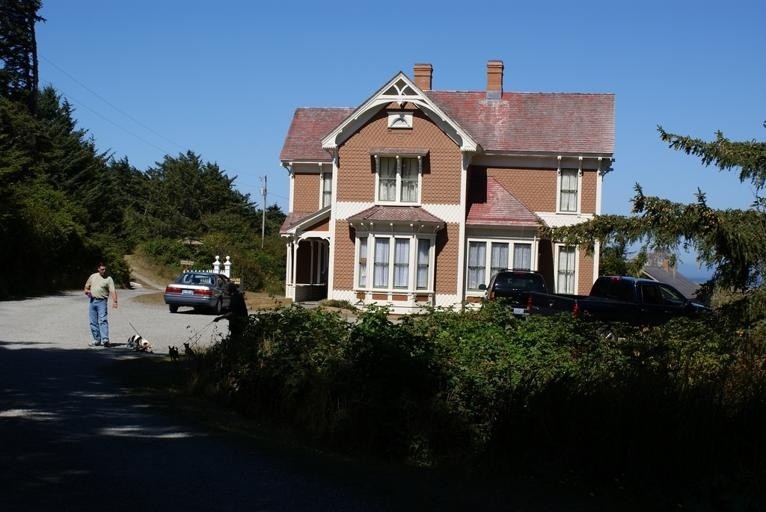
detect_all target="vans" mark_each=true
[479,271,548,318]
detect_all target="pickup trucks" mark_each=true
[526,275,714,327]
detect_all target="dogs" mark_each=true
[184,343,194,360]
[125,334,153,353]
[169,346,178,361]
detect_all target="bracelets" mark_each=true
[113,300,117,304]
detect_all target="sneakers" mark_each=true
[87,339,109,347]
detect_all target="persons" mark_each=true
[83,261,119,349]
[212,283,249,338]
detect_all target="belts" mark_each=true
[93,297,106,300]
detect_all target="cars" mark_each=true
[164,271,245,315]
